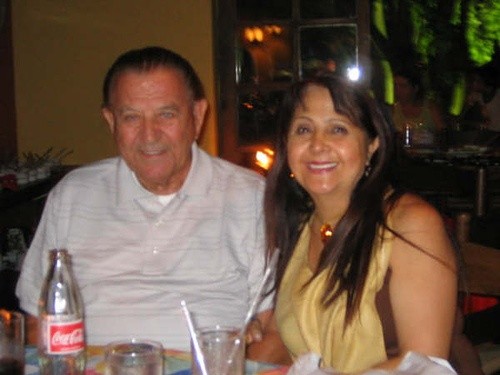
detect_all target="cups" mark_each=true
[191,326,246,375]
[0,309,24,375]
[104,339,164,374]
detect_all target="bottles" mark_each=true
[37,249,86,375]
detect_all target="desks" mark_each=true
[394,134,500,230]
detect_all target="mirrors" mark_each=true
[298,23,360,81]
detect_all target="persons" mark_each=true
[237,55,500,375]
[16,45,286,375]
